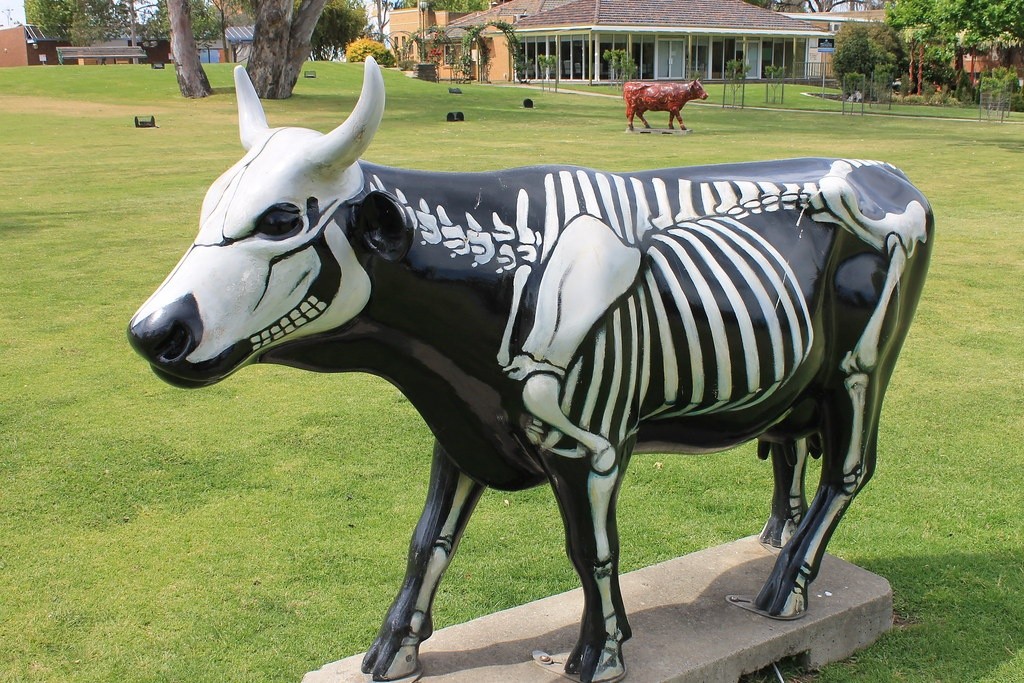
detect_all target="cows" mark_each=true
[124,56,936,682]
[623,77,708,131]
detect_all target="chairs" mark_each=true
[520,60,653,79]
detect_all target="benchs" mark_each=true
[56,46,149,64]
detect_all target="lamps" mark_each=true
[449,87,463,95]
[135,116,155,127]
[524,99,533,108]
[447,111,464,122]
[151,61,165,69]
[304,71,317,78]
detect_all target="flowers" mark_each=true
[429,31,443,57]
